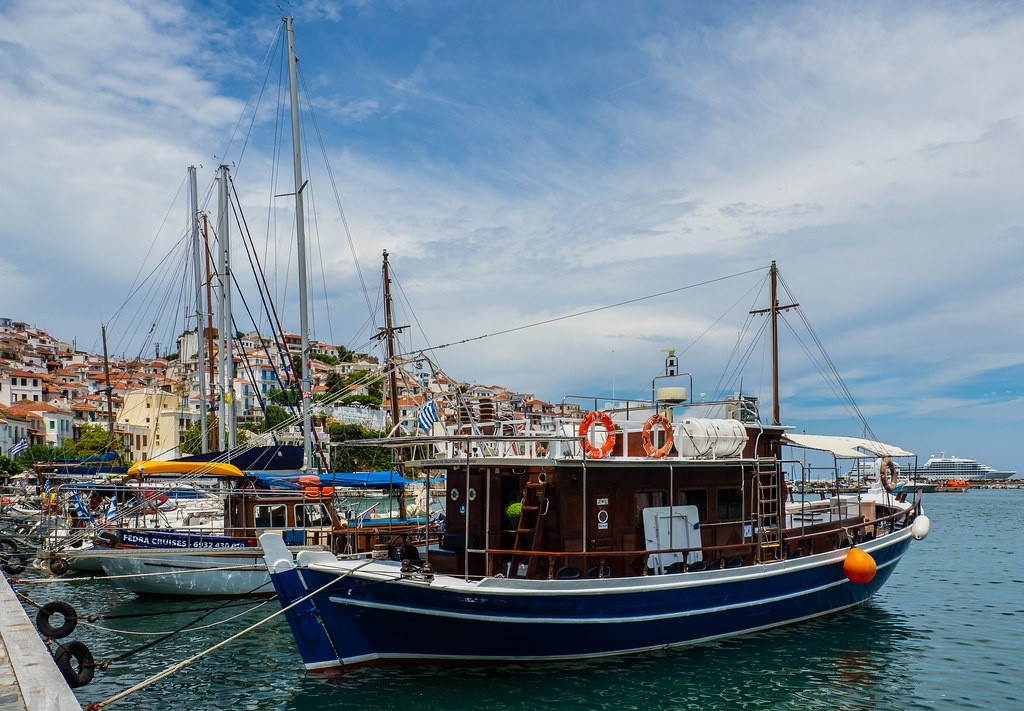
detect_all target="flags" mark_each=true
[44,479,117,519]
[10,437,30,454]
[418,400,437,432]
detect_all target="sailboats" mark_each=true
[257,251,930,672]
[99,2,446,595]
[0,154,442,575]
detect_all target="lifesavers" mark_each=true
[880,457,897,491]
[44,503,62,515]
[371,509,375,513]
[1,551,27,575]
[54,641,95,688]
[0,538,18,552]
[98,518,111,532]
[578,411,616,459]
[36,601,78,639]
[641,415,675,459]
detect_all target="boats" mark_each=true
[842,450,1016,493]
